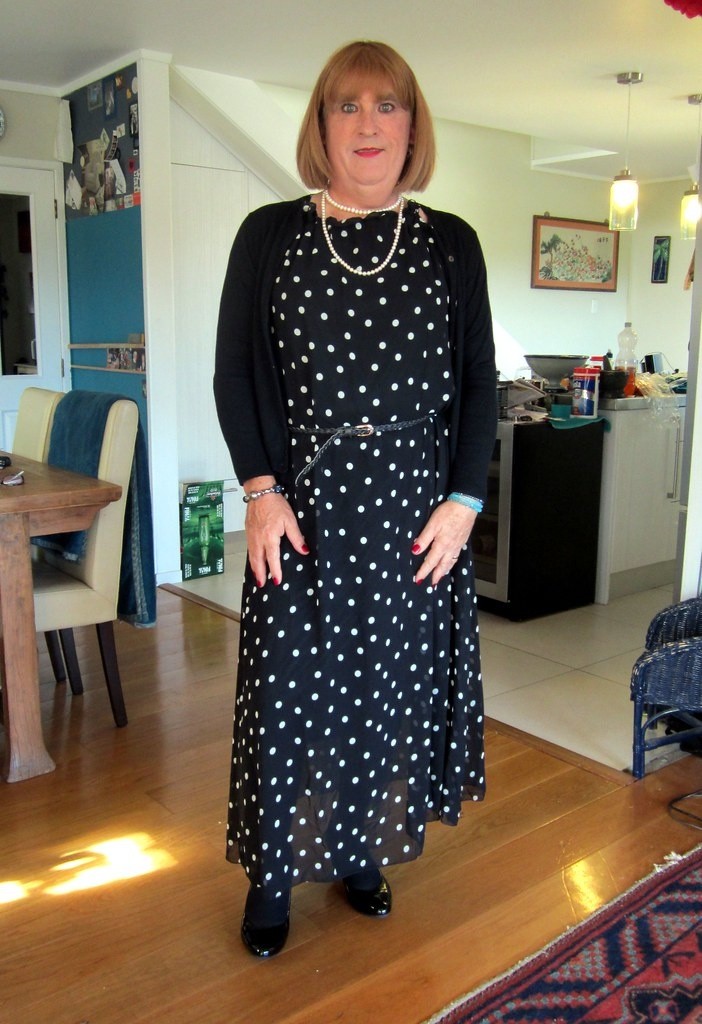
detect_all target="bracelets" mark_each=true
[448,493,485,513]
[243,484,286,503]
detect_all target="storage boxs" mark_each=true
[177,481,225,581]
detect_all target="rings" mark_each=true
[453,557,458,560]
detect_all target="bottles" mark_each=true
[615,322,637,397]
[570,356,605,419]
[199,515,211,565]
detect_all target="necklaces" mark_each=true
[322,180,405,276]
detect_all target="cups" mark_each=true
[495,386,508,420]
[551,404,572,419]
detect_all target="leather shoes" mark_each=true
[328,835,392,916]
[241,844,295,958]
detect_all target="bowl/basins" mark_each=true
[524,354,589,389]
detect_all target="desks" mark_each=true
[0,449,121,782]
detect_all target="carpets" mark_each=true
[420,847,702,1024]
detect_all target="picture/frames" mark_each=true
[531,216,619,291]
[651,235,671,283]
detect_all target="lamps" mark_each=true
[607,71,644,232]
[680,94,702,240]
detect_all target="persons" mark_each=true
[107,351,146,371]
[214,43,491,955]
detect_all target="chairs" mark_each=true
[629,596,701,779]
[0,386,140,728]
